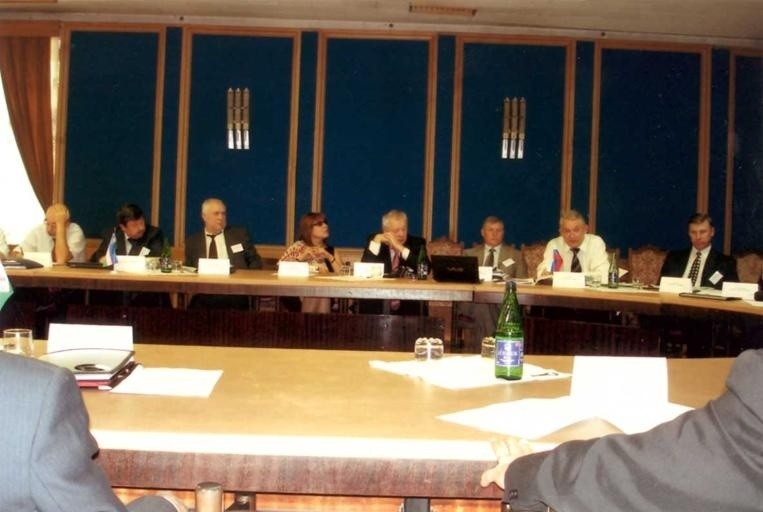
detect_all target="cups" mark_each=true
[4,328,34,360]
[171,261,180,274]
[632,276,643,290]
[480,338,496,359]
[591,276,601,290]
[340,266,351,277]
[415,336,443,361]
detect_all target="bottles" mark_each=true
[608,253,619,288]
[417,245,428,280]
[160,238,172,273]
[494,282,524,380]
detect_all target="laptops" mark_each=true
[429,254,485,286]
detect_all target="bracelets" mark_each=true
[295,258,299,261]
[330,258,335,265]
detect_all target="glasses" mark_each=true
[312,219,329,226]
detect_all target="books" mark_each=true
[38,350,137,390]
[1,256,43,269]
[496,275,552,287]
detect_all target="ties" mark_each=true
[484,248,495,267]
[392,248,401,271]
[207,234,218,259]
[688,252,702,285]
[569,247,582,273]
[52,237,57,262]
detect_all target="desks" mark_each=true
[0,265,472,354]
[0,338,737,511]
[473,280,762,318]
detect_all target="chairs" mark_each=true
[736,250,763,282]
[424,233,466,274]
[520,241,549,280]
[628,246,671,285]
[606,247,619,271]
[471,240,519,277]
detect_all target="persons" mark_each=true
[358,212,430,316]
[658,211,742,358]
[277,213,344,312]
[183,199,266,310]
[89,205,172,307]
[0,350,190,512]
[480,349,763,512]
[0,231,9,260]
[535,211,610,325]
[458,217,527,345]
[10,204,86,338]
